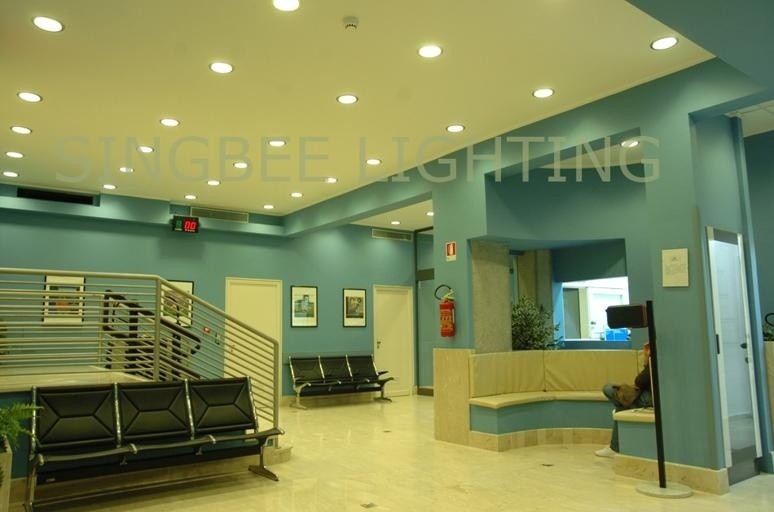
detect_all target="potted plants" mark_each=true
[0,403,44,511]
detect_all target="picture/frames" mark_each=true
[342,287,367,328]
[159,280,194,328]
[43,275,85,326]
[290,285,318,328]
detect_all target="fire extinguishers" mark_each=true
[433,284,456,337]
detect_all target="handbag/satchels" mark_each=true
[614,384,641,406]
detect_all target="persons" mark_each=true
[593,339,654,458]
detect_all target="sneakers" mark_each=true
[595,446,618,457]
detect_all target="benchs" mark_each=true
[22,376,284,511]
[468,348,656,481]
[288,352,394,409]
